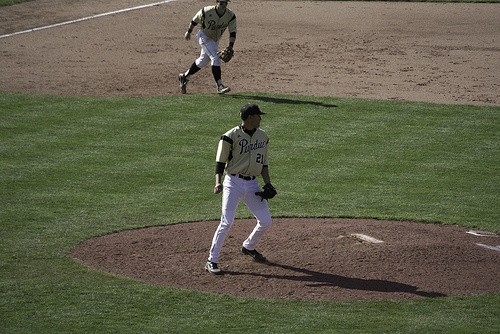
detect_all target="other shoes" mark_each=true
[217,84,231,95]
[178,72,190,94]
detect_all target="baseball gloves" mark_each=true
[218,47,234,63]
[254,183,277,200]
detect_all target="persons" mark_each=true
[178,0,237,95]
[204,103,277,275]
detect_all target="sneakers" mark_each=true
[241,247,267,262]
[204,260,223,275]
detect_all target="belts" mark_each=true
[230,173,256,181]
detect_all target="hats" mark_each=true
[239,103,267,117]
[216,0,231,3]
